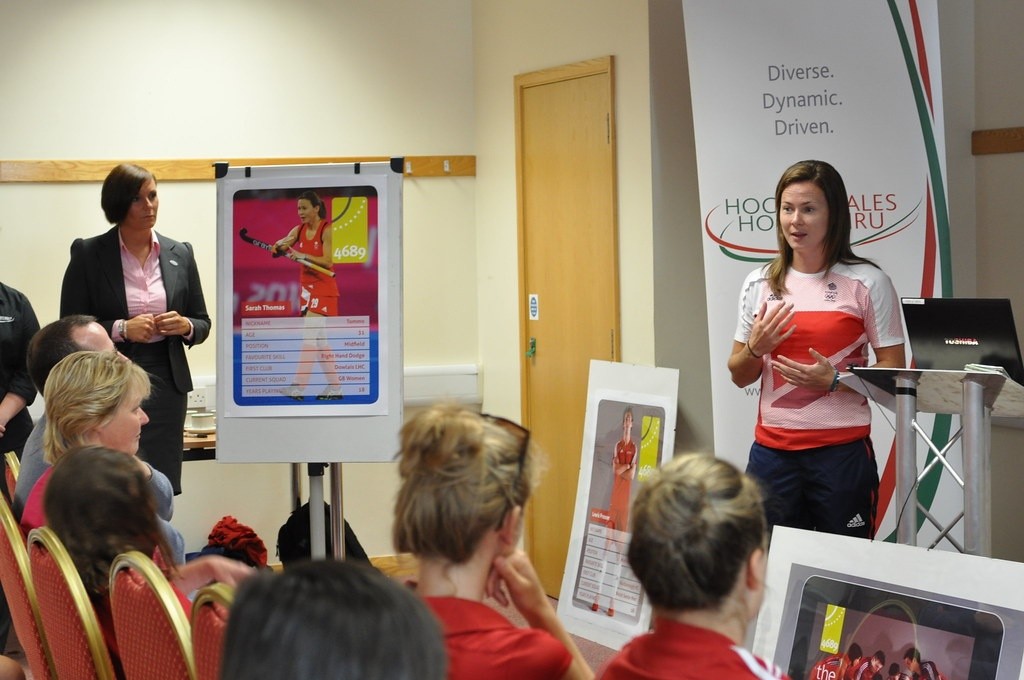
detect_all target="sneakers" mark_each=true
[317,388,344,401]
[279,385,304,401]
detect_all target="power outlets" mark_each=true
[186,388,207,408]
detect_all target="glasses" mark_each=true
[479,412,531,531]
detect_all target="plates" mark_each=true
[185,429,216,433]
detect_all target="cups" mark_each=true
[184,410,216,429]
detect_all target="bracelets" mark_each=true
[747,340,762,358]
[831,371,840,391]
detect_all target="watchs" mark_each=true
[118,319,126,341]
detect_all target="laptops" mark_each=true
[900,296,1024,386]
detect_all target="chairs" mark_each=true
[0,451,260,680]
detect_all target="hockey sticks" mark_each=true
[238,228,337,278]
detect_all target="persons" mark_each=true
[273,190,343,400]
[0,284,789,680]
[728,160,906,554]
[808,643,949,680]
[593,403,637,615]
[60,163,211,496]
